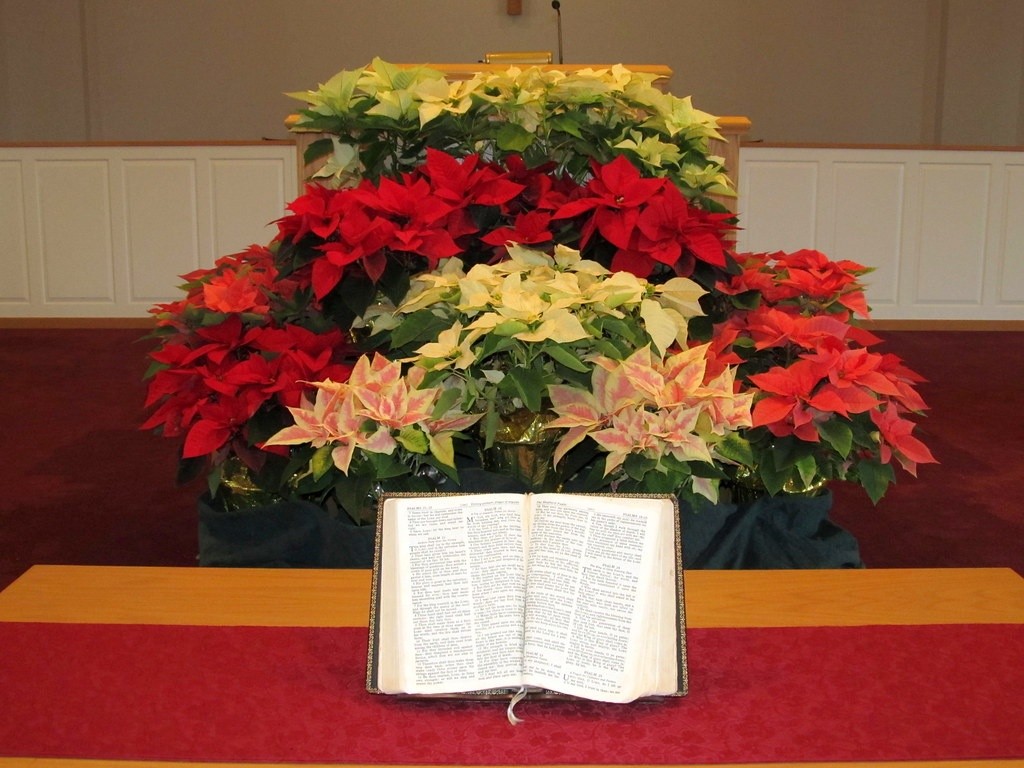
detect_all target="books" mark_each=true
[365,491,688,704]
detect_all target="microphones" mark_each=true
[551,0,563,64]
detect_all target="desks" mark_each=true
[0,566,1024,768]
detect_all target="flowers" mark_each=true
[146,59,941,523]
